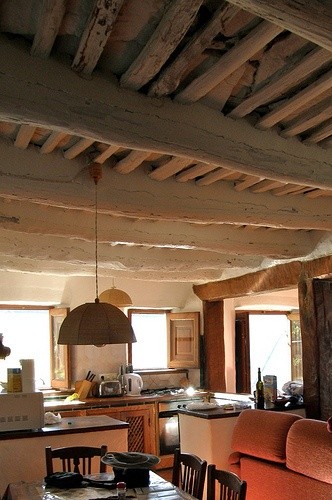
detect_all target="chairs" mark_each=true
[171,448,207,500]
[45,445,107,474]
[207,465,247,499]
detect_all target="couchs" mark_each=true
[227,409,332,500]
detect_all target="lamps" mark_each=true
[98,285,133,308]
[57,297,137,348]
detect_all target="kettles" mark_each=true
[124,373,144,397]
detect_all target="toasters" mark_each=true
[98,380,123,398]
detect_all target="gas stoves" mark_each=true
[144,386,208,411]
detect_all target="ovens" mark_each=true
[158,413,205,456]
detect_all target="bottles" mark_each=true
[116,482,126,500]
[256,367,265,408]
